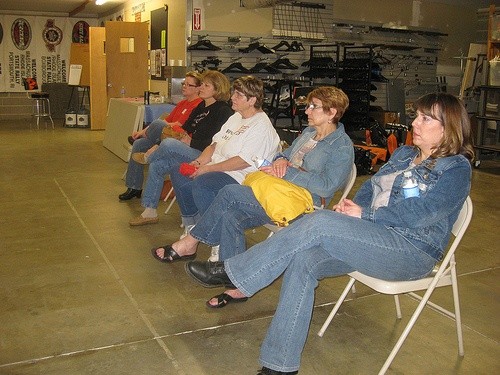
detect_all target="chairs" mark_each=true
[318,195,473,374]
[264,164,357,240]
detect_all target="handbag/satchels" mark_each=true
[241,170,314,229]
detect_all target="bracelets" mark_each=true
[195,159,201,165]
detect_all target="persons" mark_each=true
[119,71,204,201]
[23,77,38,90]
[128,71,235,225]
[167,76,283,262]
[151,87,354,263]
[184,94,475,375]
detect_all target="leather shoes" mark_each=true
[256,366,298,375]
[184,260,237,290]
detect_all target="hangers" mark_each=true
[262,79,308,120]
[390,54,434,70]
[221,54,298,72]
[186,34,221,49]
[240,39,306,52]
[301,50,391,131]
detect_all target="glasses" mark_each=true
[180,82,196,87]
[230,89,247,100]
[305,103,322,110]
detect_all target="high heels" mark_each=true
[118,188,143,201]
[128,136,137,146]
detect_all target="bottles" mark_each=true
[121,86,125,98]
[252,155,271,169]
[402,171,420,199]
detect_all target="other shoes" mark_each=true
[128,215,159,226]
[207,245,220,262]
[178,225,194,240]
[131,152,149,165]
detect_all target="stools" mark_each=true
[23,78,54,129]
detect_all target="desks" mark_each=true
[63,85,90,127]
[121,97,177,124]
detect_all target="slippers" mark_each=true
[151,244,197,264]
[206,292,248,309]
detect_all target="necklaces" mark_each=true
[420,154,423,161]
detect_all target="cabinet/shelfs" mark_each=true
[474,61,500,168]
